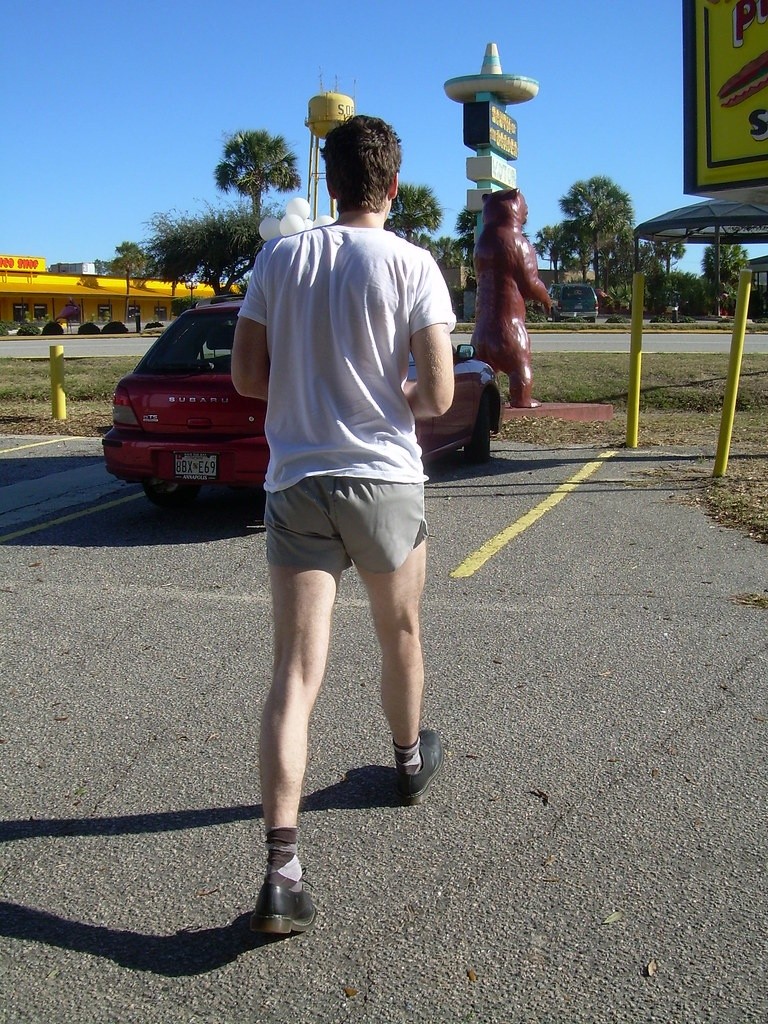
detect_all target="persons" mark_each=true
[231,115,456,937]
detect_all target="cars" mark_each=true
[101,292,507,506]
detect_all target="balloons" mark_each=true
[312,215,333,227]
[258,217,282,241]
[280,214,304,236]
[303,219,313,230]
[285,197,310,220]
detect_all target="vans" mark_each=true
[546,282,599,323]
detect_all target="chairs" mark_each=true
[198,325,235,374]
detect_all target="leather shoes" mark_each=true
[249,867,317,934]
[394,729,443,805]
